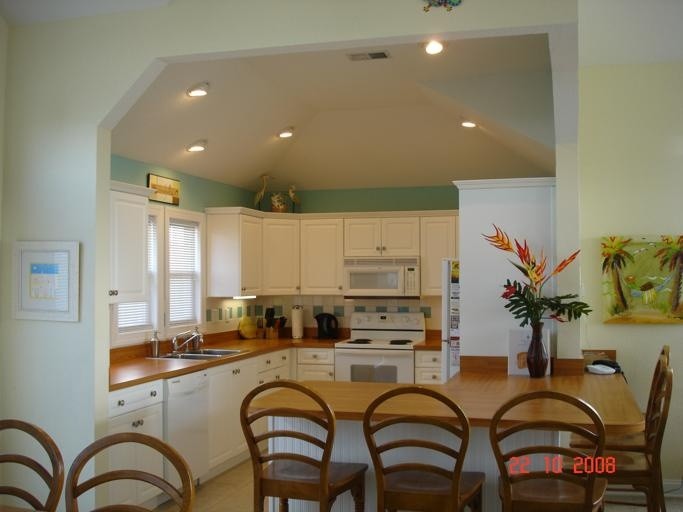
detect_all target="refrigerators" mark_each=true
[438,258,459,383]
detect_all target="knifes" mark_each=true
[264,309,287,328]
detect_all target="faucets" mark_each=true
[178,333,201,349]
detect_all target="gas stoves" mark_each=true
[333,336,423,351]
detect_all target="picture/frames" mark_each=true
[9,238,80,322]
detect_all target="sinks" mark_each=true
[144,352,222,361]
[180,348,241,357]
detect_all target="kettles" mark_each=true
[313,313,339,340]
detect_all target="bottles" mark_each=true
[149,330,160,358]
[290,305,303,339]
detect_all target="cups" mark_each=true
[255,328,265,338]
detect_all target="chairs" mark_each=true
[560,344,674,511]
[362,384,486,512]
[488,389,608,512]
[65,432,196,512]
[0,418,65,511]
[240,379,368,512]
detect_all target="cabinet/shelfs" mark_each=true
[415,350,442,385]
[344,218,421,258]
[420,217,460,297]
[253,348,291,446]
[297,347,334,382]
[164,369,207,494]
[206,358,253,473]
[263,217,344,297]
[110,190,152,305]
[206,214,263,302]
[109,379,164,505]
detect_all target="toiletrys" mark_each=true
[191,326,202,350]
[151,330,161,356]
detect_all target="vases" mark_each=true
[526,322,548,378]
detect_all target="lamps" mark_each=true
[187,140,208,152]
[426,41,443,54]
[188,81,208,97]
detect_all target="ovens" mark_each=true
[334,347,414,385]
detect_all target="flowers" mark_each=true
[477,222,592,327]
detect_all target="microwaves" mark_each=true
[341,256,420,298]
